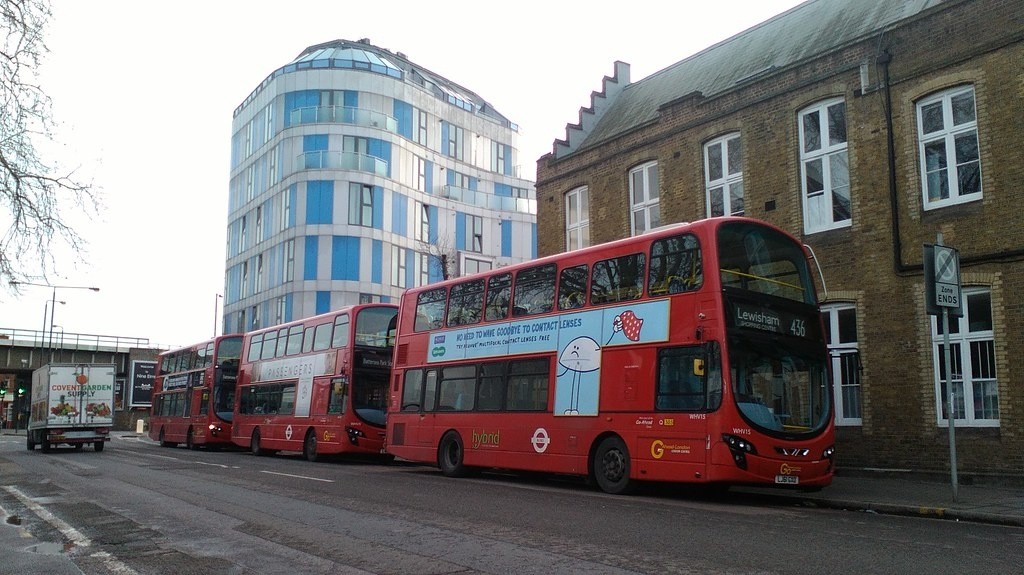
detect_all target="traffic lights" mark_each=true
[0,381,5,395]
[18,381,24,396]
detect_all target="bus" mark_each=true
[388,216,865,495]
[232,303,431,463]
[148,333,245,451]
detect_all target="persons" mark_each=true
[2,415,6,428]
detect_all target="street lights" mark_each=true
[53,325,64,364]
[9,280,99,364]
[40,299,65,367]
[215,293,222,339]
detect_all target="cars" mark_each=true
[0,416,3,429]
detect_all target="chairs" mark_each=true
[404,391,763,411]
[429,276,691,331]
[243,398,381,414]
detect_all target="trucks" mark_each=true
[26,363,116,453]
